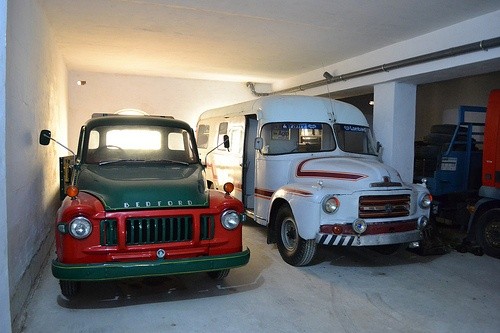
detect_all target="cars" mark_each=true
[38,112,251,298]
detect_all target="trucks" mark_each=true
[408,88,500,259]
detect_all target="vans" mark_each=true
[194,94,433,266]
